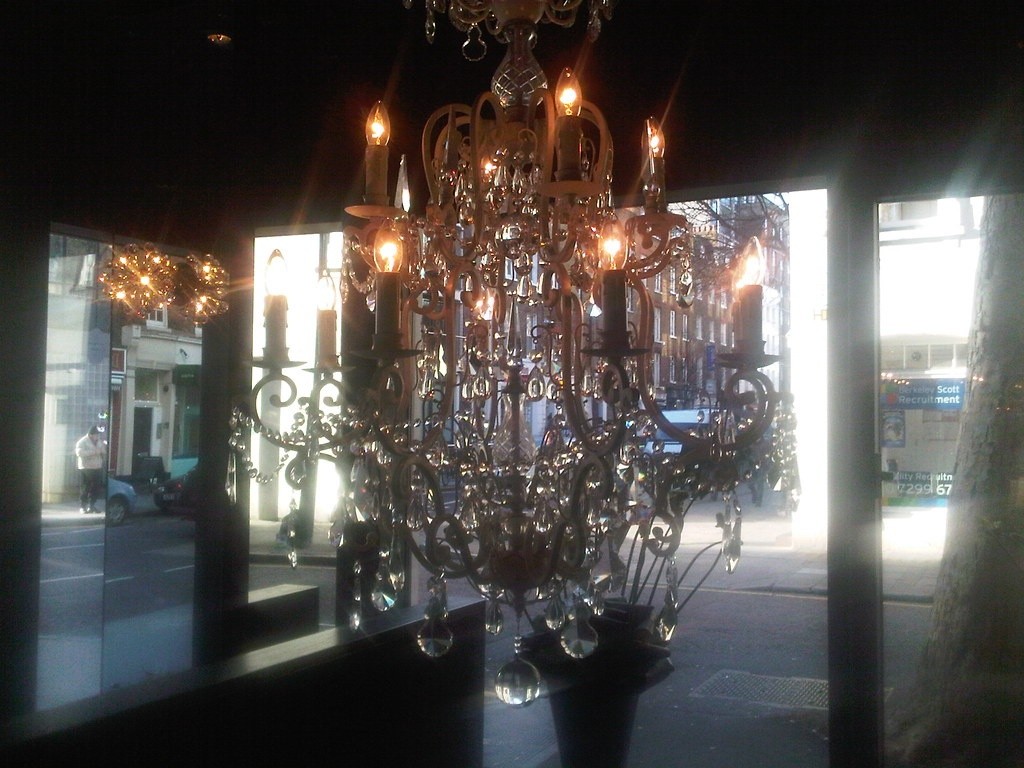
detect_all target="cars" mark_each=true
[154,462,198,514]
[107,479,136,525]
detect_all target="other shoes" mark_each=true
[80,507,87,514]
[87,507,101,513]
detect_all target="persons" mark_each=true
[75,426,107,514]
[745,435,768,507]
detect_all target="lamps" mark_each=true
[226,0,781,710]
[89,241,230,326]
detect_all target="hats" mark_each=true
[89,425,99,434]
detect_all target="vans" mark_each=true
[644,409,717,457]
